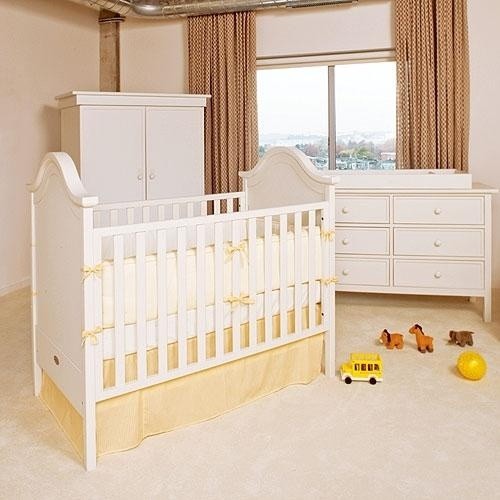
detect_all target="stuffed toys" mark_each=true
[447,330,475,348]
[379,329,405,350]
[409,324,434,353]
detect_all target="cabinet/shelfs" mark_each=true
[55,91,212,229]
[335,181,500,321]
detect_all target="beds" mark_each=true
[25,145,340,473]
